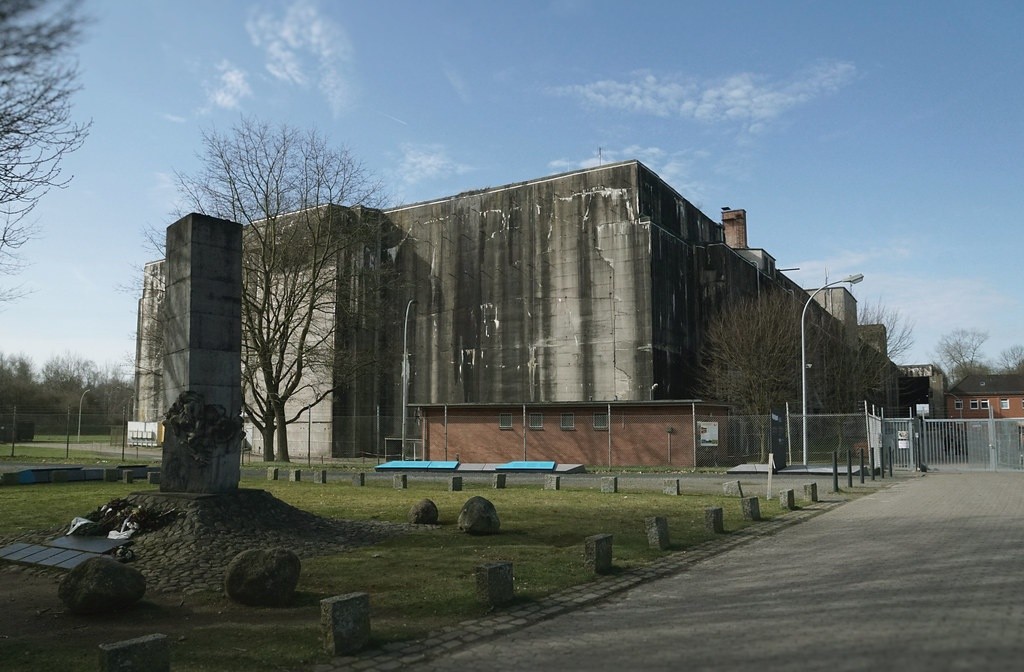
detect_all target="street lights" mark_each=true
[801,273,864,466]
[78,388,91,443]
[401,300,419,461]
